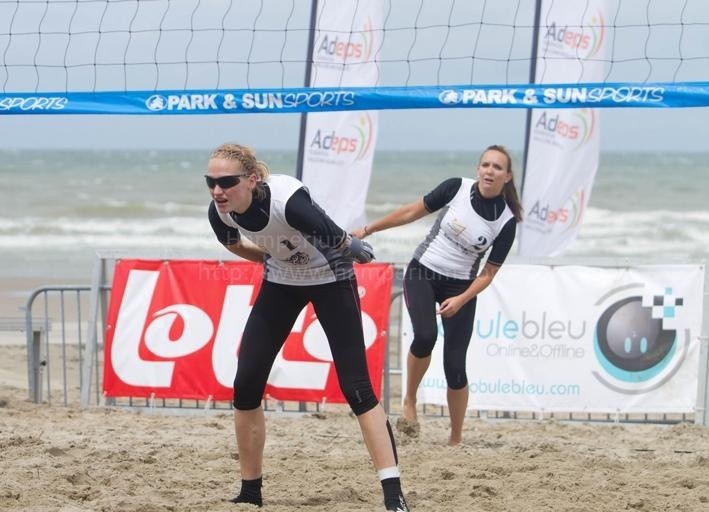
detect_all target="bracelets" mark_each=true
[363,225,370,237]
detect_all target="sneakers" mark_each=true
[230,495,263,507]
[382,495,409,512]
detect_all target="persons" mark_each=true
[346,141,524,449]
[201,143,413,512]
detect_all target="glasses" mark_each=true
[206,174,249,190]
